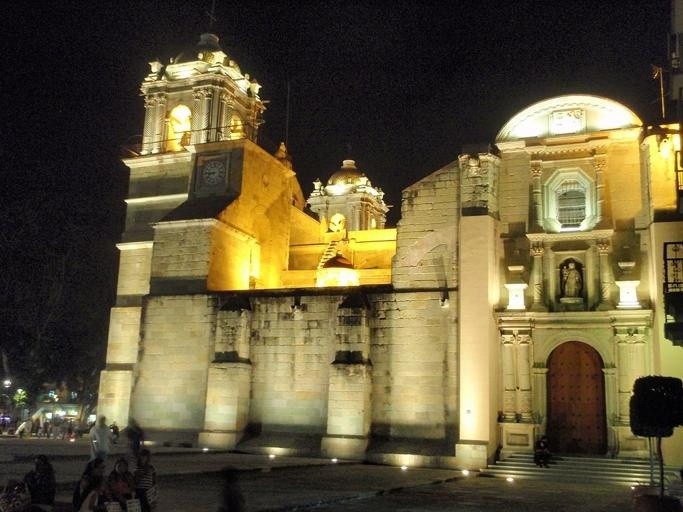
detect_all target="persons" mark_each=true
[0,403,156,511]
[533,442,549,469]
[560,260,582,297]
[535,436,555,456]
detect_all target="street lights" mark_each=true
[51,393,59,422]
[15,386,25,430]
[3,379,12,416]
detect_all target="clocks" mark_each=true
[202,160,226,186]
